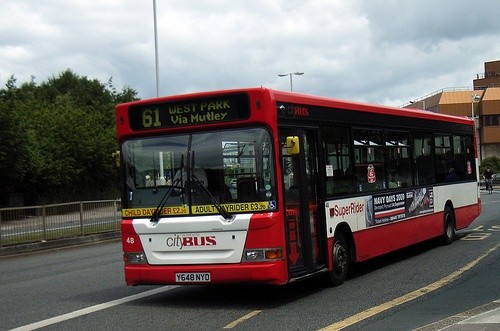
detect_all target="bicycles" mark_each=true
[485,177,493,194]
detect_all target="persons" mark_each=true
[482,167,494,191]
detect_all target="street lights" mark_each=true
[278,73,304,92]
[471,93,480,118]
[409,100,425,111]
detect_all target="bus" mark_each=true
[115,87,482,286]
